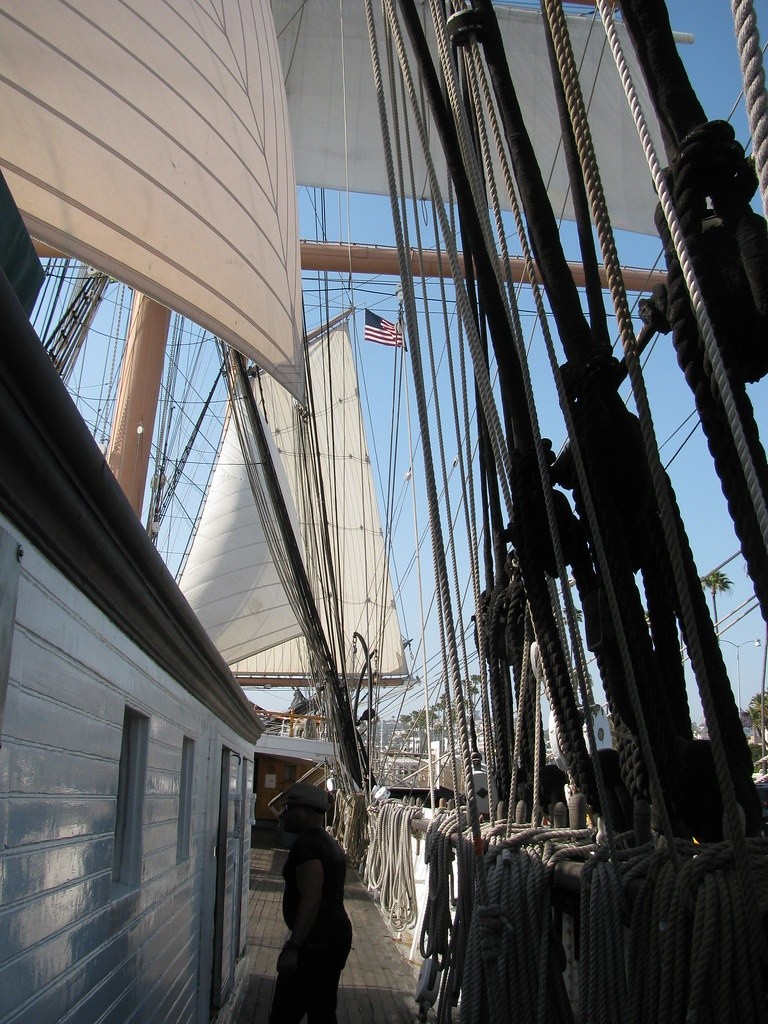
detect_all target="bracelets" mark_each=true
[284,941,299,951]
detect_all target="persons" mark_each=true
[268,783,353,1024]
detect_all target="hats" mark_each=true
[281,782,331,809]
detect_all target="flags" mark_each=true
[366,309,408,352]
